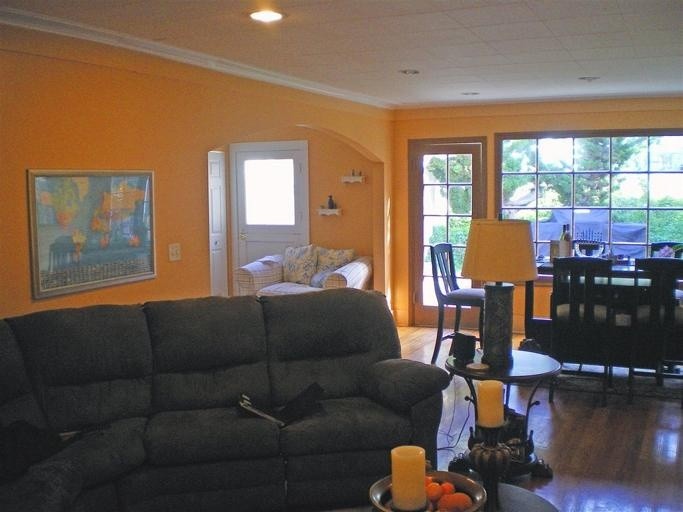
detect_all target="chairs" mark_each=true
[525,240,682,407]
[430,242,486,365]
[233,254,375,297]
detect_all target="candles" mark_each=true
[391,445,426,510]
[476,379,505,427]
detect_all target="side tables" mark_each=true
[446,349,563,478]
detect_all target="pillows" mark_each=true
[282,244,353,287]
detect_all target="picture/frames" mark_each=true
[27,170,156,300]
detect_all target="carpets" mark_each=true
[505,363,682,399]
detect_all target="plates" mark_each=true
[367,466,487,512]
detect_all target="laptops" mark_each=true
[238,381,326,428]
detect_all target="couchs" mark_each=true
[0,288,451,511]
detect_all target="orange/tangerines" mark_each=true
[426,476,471,512]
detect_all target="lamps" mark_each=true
[461,219,539,369]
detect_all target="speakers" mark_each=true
[448,331,476,365]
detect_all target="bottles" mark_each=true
[557,223,573,256]
